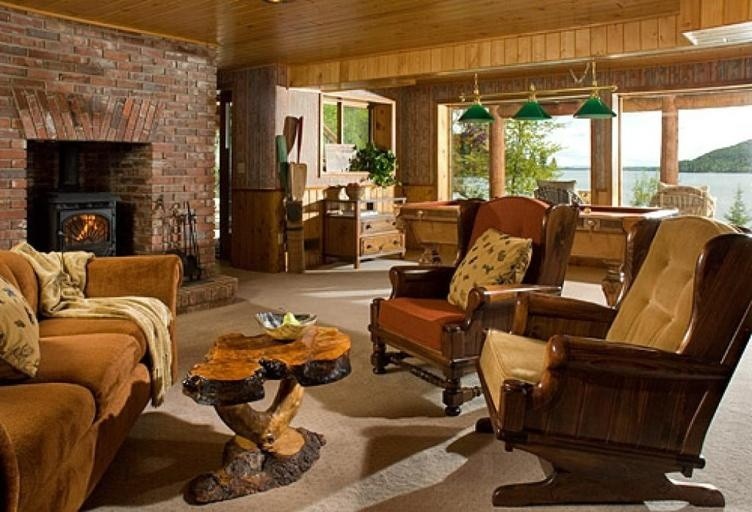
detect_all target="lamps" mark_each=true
[512,79,553,122]
[573,58,617,120]
[458,72,495,124]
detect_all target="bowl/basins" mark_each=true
[252,310,320,341]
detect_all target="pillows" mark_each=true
[446,227,532,308]
[0,280,42,375]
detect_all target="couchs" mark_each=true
[0,251,185,512]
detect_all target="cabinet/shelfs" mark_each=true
[321,198,409,270]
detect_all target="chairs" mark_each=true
[368,196,580,416]
[650,182,718,220]
[535,179,591,207]
[475,215,752,508]
